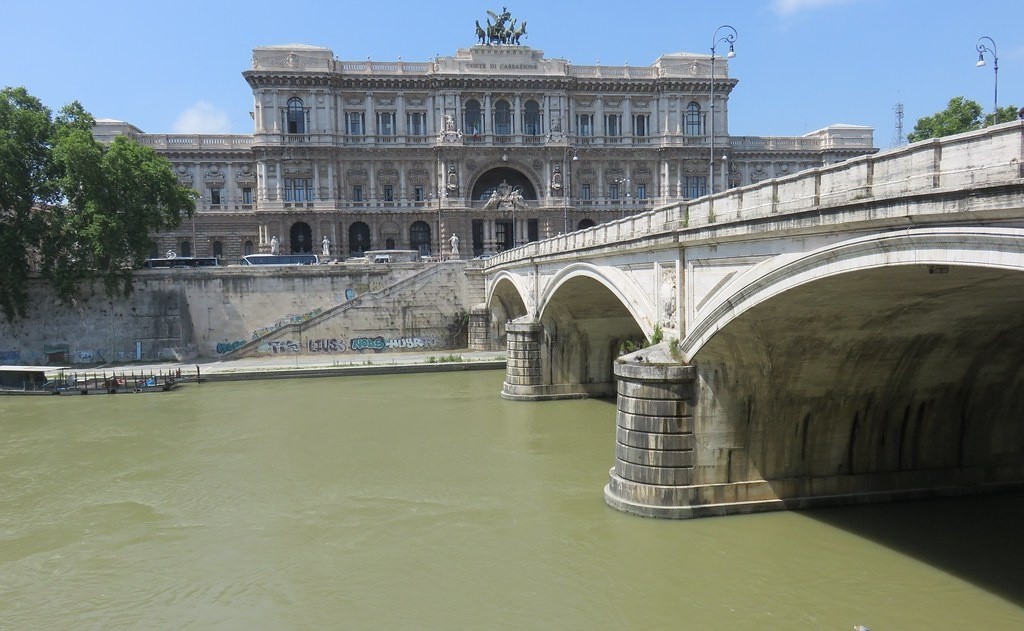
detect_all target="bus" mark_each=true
[240,253,319,267]
[140,257,220,269]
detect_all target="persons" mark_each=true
[448,233,458,247]
[322,236,330,255]
[486,179,523,205]
[271,236,280,255]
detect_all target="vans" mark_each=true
[373,255,393,263]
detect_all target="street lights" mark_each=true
[563,149,581,234]
[438,184,459,262]
[511,189,523,248]
[709,24,738,193]
[976,36,999,125]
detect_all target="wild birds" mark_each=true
[854,624,871,631]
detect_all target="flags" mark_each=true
[473,123,478,142]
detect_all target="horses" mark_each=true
[473,6,529,45]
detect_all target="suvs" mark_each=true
[472,254,491,259]
